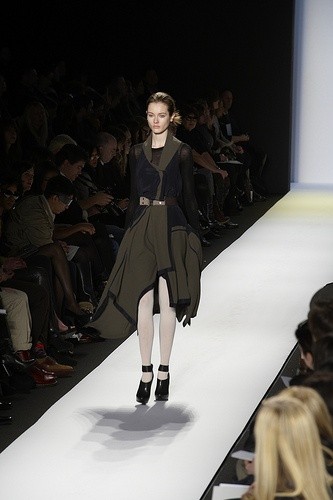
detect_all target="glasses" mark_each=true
[1,190,19,200]
[184,116,197,122]
[57,195,73,206]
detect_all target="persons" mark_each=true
[210,282,333,500]
[88,91,208,404]
[0,38,266,431]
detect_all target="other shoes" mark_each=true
[203,189,266,246]
[0,301,106,422]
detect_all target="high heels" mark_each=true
[136,364,154,404]
[155,364,170,400]
